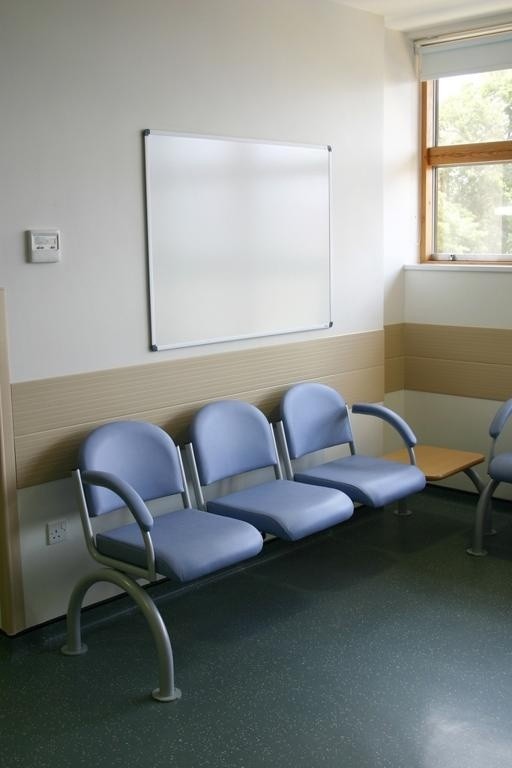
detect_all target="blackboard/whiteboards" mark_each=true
[137,129,336,355]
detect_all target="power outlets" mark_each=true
[47,518,69,546]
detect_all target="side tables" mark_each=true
[378,445,498,538]
